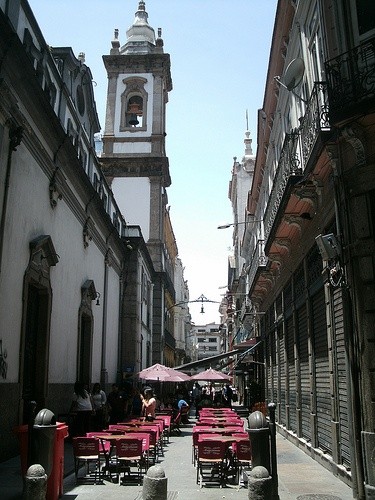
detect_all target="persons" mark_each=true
[106,383,125,424]
[68,381,93,431]
[91,382,108,430]
[123,379,238,424]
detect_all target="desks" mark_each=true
[156,413,168,416]
[117,423,158,427]
[104,427,149,433]
[210,410,227,414]
[206,418,230,422]
[201,422,240,428]
[96,434,133,484]
[203,436,249,485]
[200,428,243,435]
[139,417,162,421]
[214,414,227,418]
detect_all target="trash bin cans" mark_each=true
[17,408,70,500]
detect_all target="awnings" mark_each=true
[228,336,260,376]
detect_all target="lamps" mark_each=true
[87,292,101,306]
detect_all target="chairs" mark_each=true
[72,407,251,488]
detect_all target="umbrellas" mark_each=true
[131,362,191,385]
[191,366,234,382]
